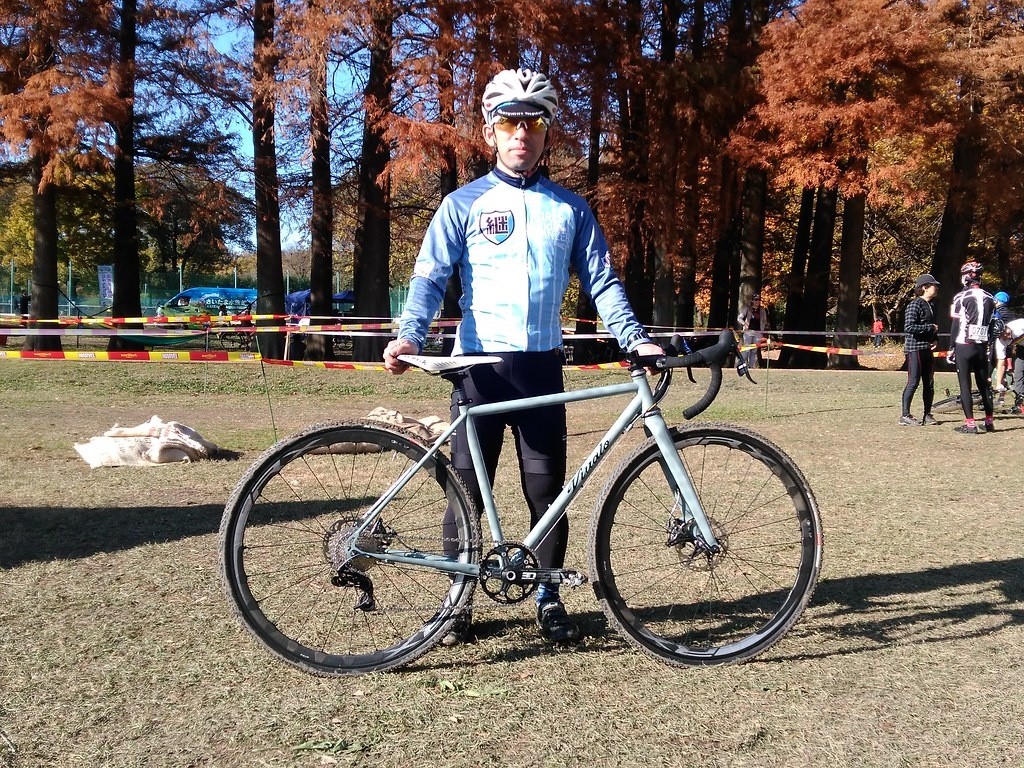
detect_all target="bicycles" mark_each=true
[334,317,445,353]
[931,377,1024,416]
[217,333,825,680]
[220,324,259,352]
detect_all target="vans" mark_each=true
[155,286,257,318]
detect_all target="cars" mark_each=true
[285,287,354,338]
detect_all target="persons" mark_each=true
[240,302,251,316]
[218,301,228,316]
[156,302,165,317]
[18,289,31,325]
[290,334,306,360]
[177,296,188,306]
[873,316,885,348]
[10,296,20,309]
[737,294,770,368]
[100,302,109,317]
[383,64,664,647]
[988,318,1024,414]
[898,274,940,426]
[946,261,996,433]
[987,292,1009,394]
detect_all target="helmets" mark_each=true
[995,292,1009,303]
[961,262,983,274]
[481,68,559,124]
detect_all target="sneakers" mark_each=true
[536,598,579,643]
[923,414,938,425]
[899,415,922,426]
[439,609,472,646]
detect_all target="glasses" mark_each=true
[491,115,548,130]
[753,298,761,301]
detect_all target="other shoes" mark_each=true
[985,417,995,431]
[997,385,1007,393]
[964,418,977,433]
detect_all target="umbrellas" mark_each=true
[333,289,353,303]
[284,289,311,303]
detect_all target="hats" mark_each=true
[493,102,545,118]
[916,274,941,286]
[752,294,760,299]
